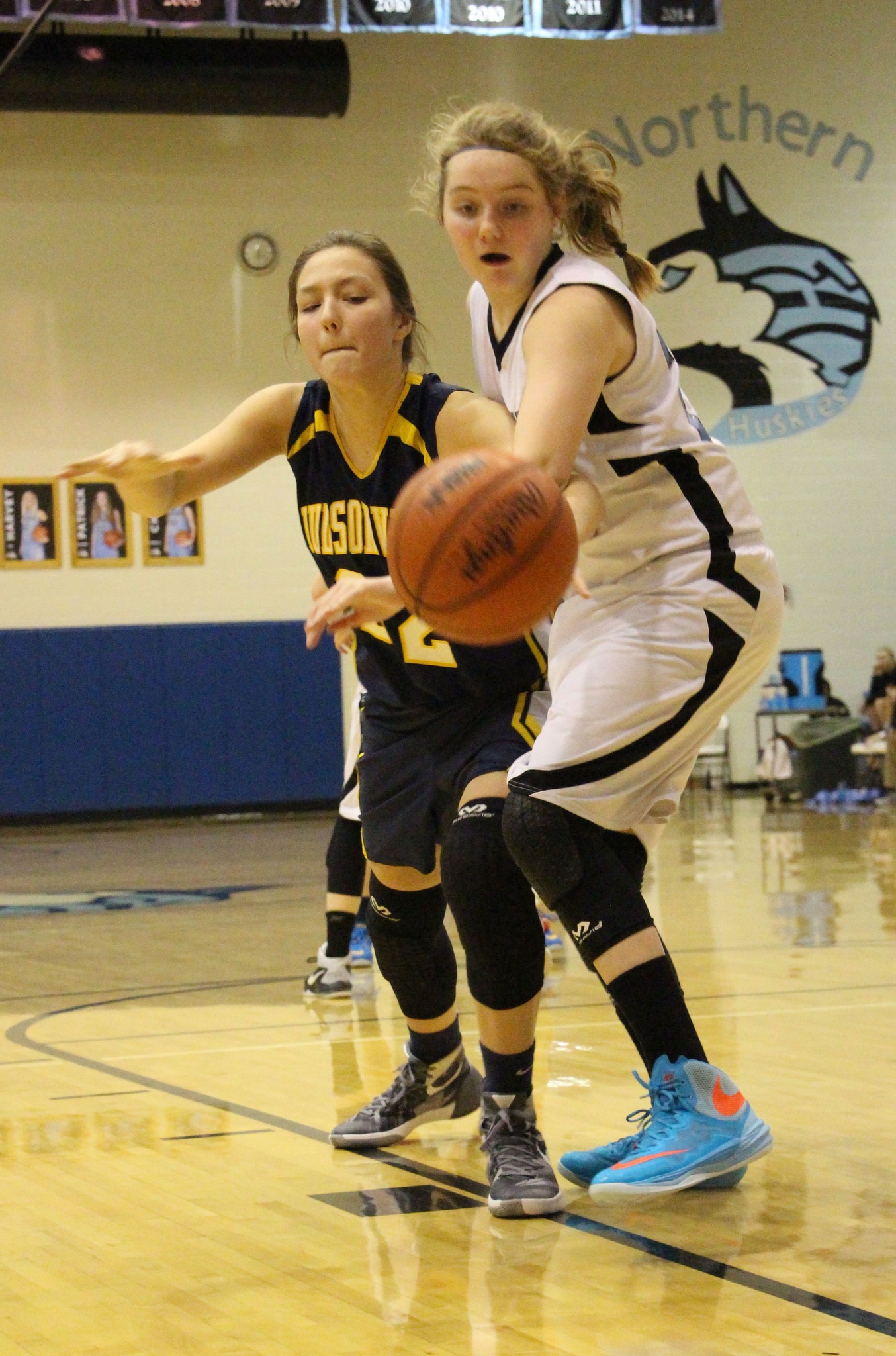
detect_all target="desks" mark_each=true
[756,710,842,804]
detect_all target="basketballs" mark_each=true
[385,446,581,647]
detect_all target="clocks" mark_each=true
[237,231,279,276]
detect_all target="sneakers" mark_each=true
[557,1118,747,1189]
[489,1220,560,1295]
[306,996,356,1039]
[328,1039,484,1148]
[351,966,376,1002]
[476,1075,561,1217]
[538,910,562,950]
[349,926,373,968]
[303,944,353,999]
[335,1132,485,1217]
[563,1195,764,1255]
[588,1053,772,1207]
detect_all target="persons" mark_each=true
[18,490,48,560]
[48,229,601,1218]
[162,505,194,555]
[304,95,778,1205]
[90,490,124,559]
[303,569,577,1000]
[761,647,896,799]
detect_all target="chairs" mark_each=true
[687,716,734,792]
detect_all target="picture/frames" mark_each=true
[0,478,60,569]
[140,497,206,566]
[67,476,135,567]
